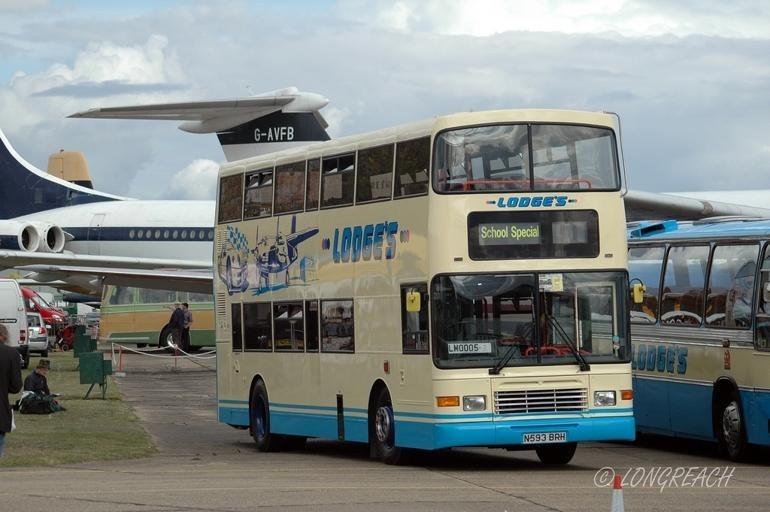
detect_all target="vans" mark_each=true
[0,279,30,368]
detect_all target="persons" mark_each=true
[22,357,51,395]
[1,319,25,464]
[179,302,193,352]
[166,301,184,357]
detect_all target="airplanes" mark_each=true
[0,87,769,293]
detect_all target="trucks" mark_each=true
[22,287,66,325]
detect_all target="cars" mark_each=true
[27,311,49,356]
[57,324,79,352]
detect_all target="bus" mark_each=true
[629,216,770,463]
[98,285,217,350]
[217,110,634,465]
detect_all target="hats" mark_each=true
[37,359,51,369]
